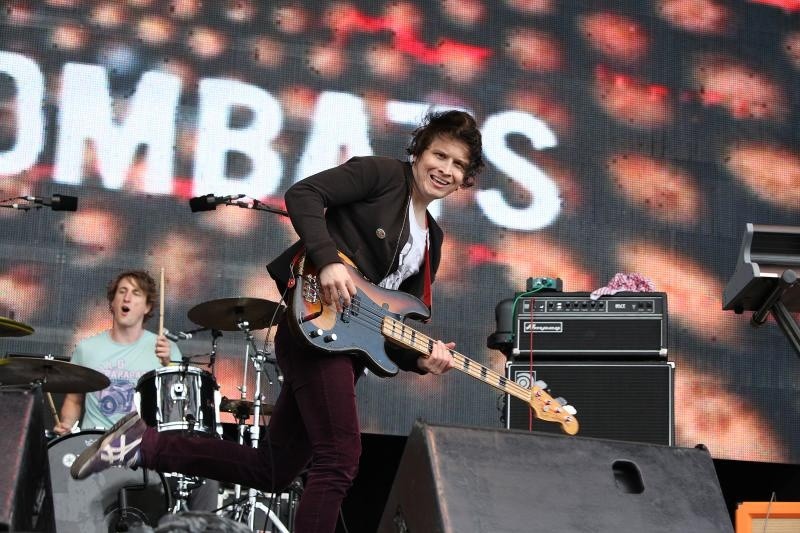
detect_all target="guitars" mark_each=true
[287,249,581,435]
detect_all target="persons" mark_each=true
[70,106,485,533]
[52,267,188,436]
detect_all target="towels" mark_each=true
[589,273,652,301]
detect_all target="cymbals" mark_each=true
[0,316,36,338]
[219,397,276,416]
[188,297,285,331]
[0,358,111,394]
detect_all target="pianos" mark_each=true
[720,222,800,354]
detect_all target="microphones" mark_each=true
[163,328,178,342]
[190,193,245,212]
[24,194,77,212]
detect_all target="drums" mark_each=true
[47,428,173,533]
[136,360,223,440]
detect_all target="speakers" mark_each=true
[505,361,676,446]
[376,417,735,533]
